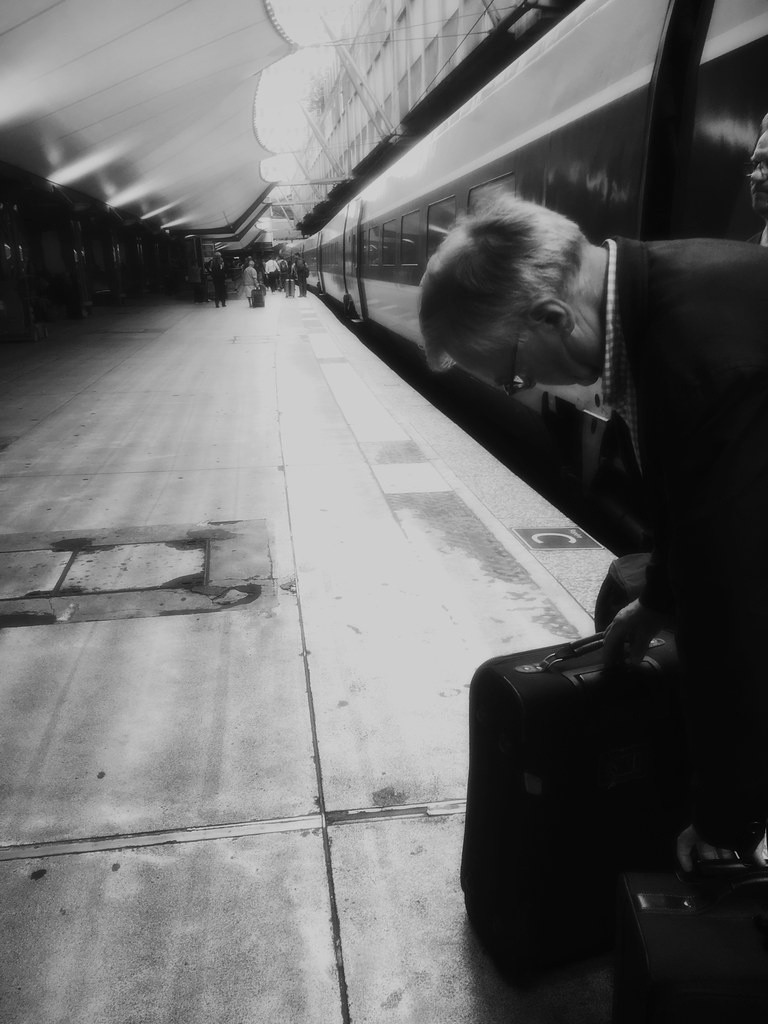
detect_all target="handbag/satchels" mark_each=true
[303,260,309,278]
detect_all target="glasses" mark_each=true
[503,317,534,395]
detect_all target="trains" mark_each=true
[280,0,768,486]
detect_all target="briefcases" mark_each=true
[611,860,768,1024]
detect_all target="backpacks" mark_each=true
[279,259,287,272]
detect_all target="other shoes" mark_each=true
[272,291,275,294]
[216,306,219,308]
[222,305,227,307]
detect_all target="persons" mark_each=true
[746,114,767,246]
[414,197,768,875]
[212,247,310,307]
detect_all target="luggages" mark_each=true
[285,279,295,298]
[251,286,264,307]
[462,627,697,990]
